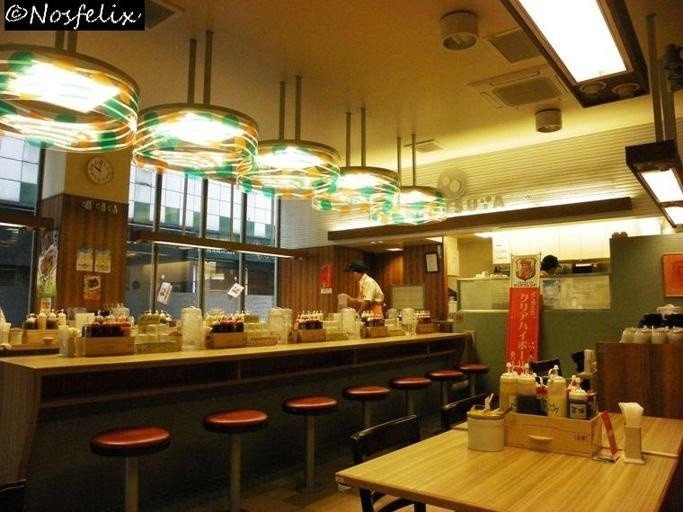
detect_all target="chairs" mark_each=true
[528,358,563,387]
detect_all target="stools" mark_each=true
[90,363,490,512]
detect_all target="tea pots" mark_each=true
[621,325,683,344]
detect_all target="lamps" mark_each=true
[0,0,447,227]
[439,13,479,52]
[499,0,682,232]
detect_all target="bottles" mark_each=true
[296,309,385,330]
[499,363,588,420]
[81,309,134,338]
[211,313,244,333]
[21,308,67,330]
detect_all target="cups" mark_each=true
[337,292,348,309]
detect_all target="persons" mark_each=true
[341,261,384,326]
[539,255,560,293]
[518,261,535,280]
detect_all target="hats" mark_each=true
[542,254,563,268]
[343,263,366,273]
